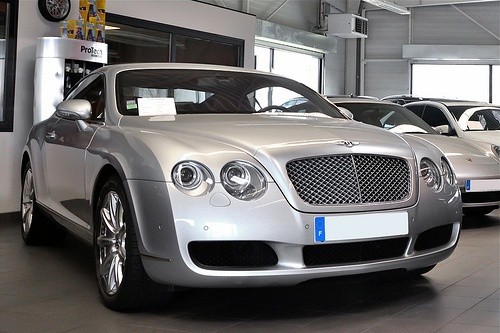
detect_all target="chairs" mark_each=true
[206,88,253,114]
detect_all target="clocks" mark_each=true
[38,0,71,22]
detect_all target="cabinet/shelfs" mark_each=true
[33,37,108,126]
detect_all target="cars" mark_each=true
[18,62,464,313]
[402,99,500,165]
[276,99,500,215]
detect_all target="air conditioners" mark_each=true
[328,14,369,38]
